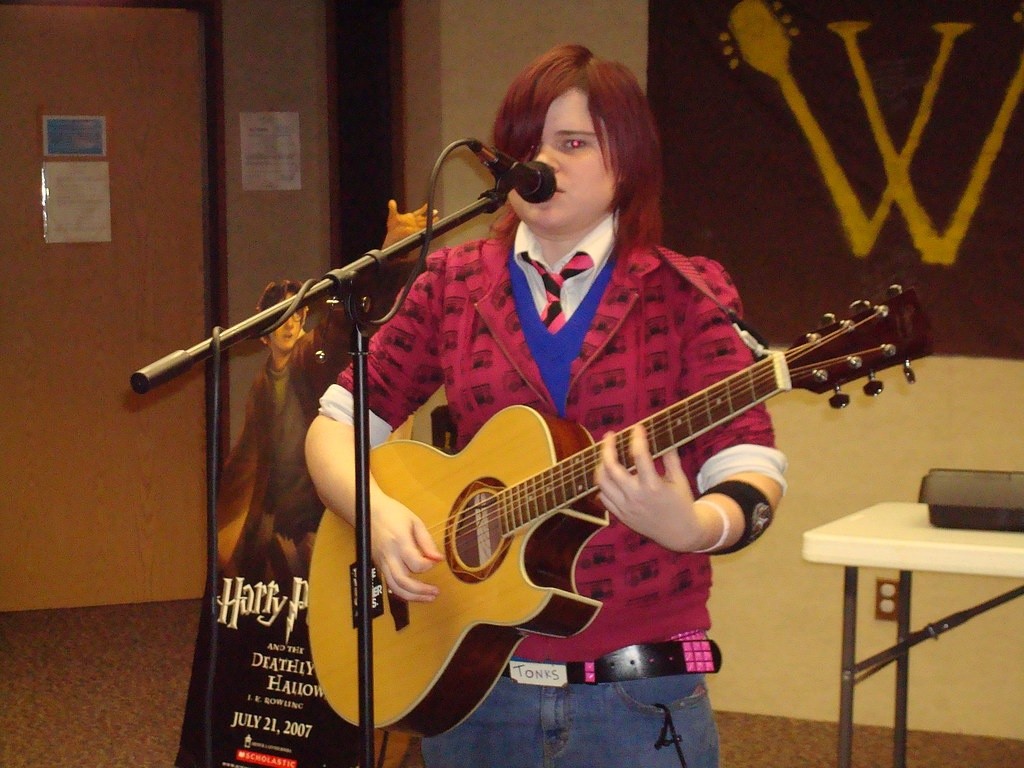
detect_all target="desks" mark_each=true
[802,502,1024,768]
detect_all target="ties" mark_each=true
[522,251,594,334]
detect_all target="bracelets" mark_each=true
[693,501,730,552]
[702,480,772,555]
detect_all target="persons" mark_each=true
[304,44,787,768]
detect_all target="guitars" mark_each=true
[304,278,941,741]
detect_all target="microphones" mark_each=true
[464,138,556,202]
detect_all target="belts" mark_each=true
[503,631,724,687]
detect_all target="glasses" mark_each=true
[293,309,302,322]
